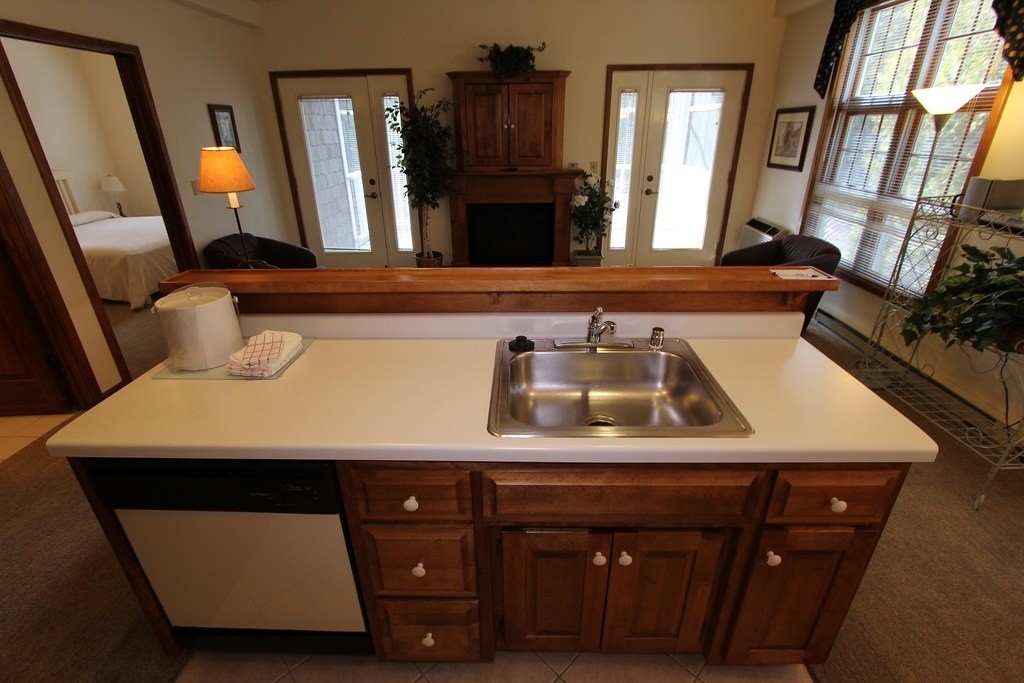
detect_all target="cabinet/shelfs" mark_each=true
[444,70,572,168]
[332,460,911,665]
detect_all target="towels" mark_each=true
[225,329,303,377]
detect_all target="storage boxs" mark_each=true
[955,176,1024,224]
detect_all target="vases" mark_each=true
[574,249,603,268]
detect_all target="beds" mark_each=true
[55,180,180,310]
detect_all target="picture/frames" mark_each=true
[766,105,816,172]
[207,103,241,153]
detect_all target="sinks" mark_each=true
[486,350,755,438]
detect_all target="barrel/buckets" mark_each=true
[152,285,245,372]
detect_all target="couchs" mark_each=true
[720,234,840,333]
[204,233,318,270]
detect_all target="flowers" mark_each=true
[569,167,620,255]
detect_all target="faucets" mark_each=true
[587,306,617,344]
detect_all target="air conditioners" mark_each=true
[736,217,790,250]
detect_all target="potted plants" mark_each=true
[384,88,470,269]
[898,244,1024,354]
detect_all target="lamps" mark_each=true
[99,173,128,216]
[197,146,259,269]
[911,1,986,131]
